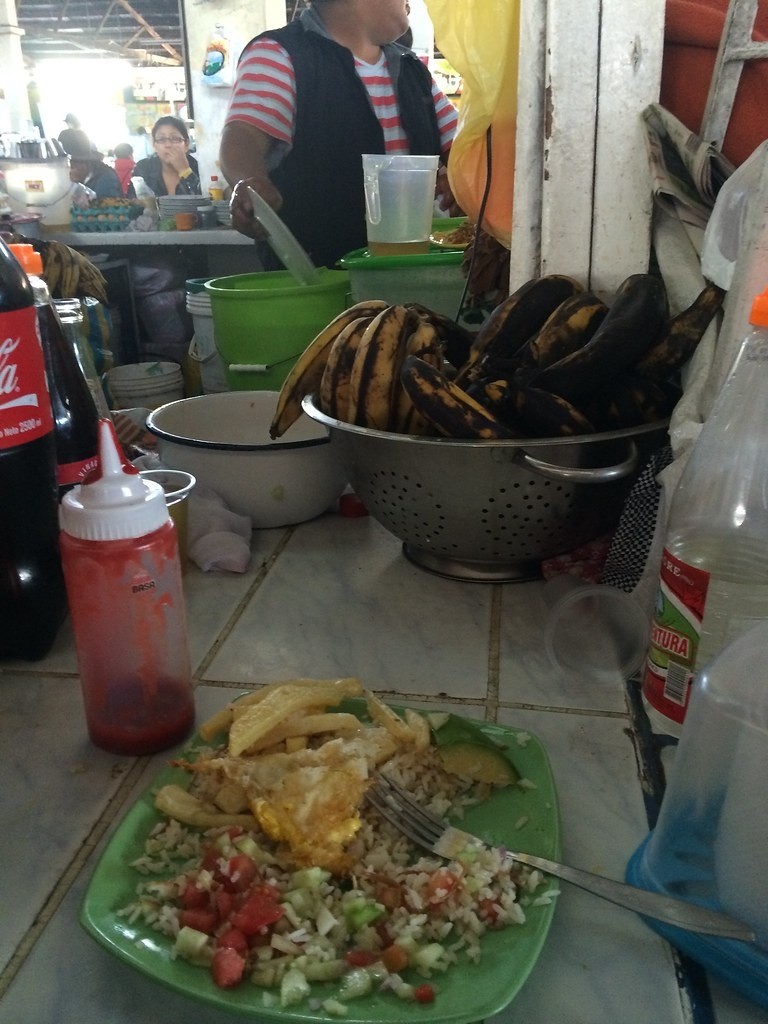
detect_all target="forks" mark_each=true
[361,766,760,944]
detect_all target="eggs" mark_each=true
[76,214,124,222]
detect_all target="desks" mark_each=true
[42,224,255,265]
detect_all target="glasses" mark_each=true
[154,135,186,143]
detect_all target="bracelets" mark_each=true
[180,167,192,179]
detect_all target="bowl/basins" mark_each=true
[8,212,42,233]
[147,392,341,527]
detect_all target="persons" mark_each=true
[57,114,200,222]
[219,1,468,272]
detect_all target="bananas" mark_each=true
[12,234,108,305]
[271,270,728,441]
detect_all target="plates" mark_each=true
[77,699,560,1024]
[426,228,474,249]
[156,194,232,227]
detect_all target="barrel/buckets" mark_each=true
[184,282,223,392]
[102,360,182,408]
[0,153,73,233]
[205,268,350,392]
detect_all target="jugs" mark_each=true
[360,156,441,252]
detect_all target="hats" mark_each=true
[58,129,104,161]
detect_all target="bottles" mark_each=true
[209,175,224,201]
[198,206,216,232]
[640,285,768,741]
[0,235,198,759]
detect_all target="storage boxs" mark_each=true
[335,216,476,323]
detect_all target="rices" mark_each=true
[119,734,560,1016]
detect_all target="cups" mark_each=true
[540,577,648,685]
[175,211,198,229]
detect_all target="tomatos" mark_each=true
[176,848,290,986]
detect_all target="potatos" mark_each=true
[157,674,434,829]
